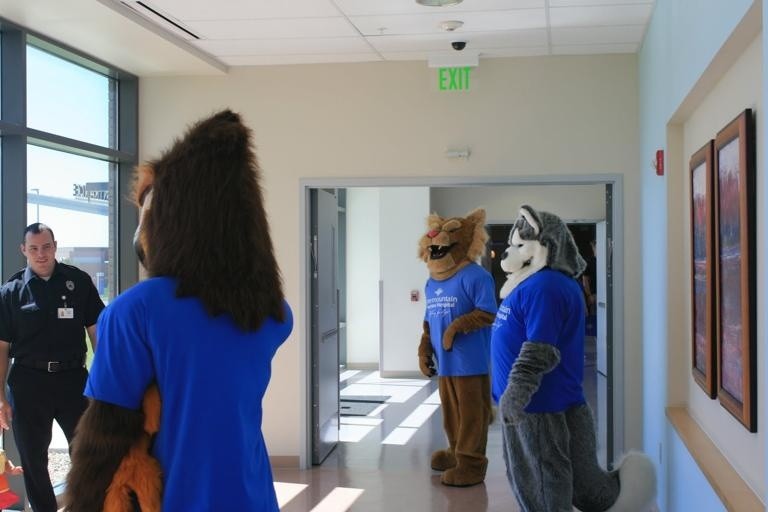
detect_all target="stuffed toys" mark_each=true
[490,204,657,512]
[61,109,294,512]
[416,209,498,487]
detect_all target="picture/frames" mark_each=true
[711,106,757,432]
[687,137,719,399]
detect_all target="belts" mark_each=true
[14,360,84,373]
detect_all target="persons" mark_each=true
[0,223,107,512]
[0,449,24,512]
